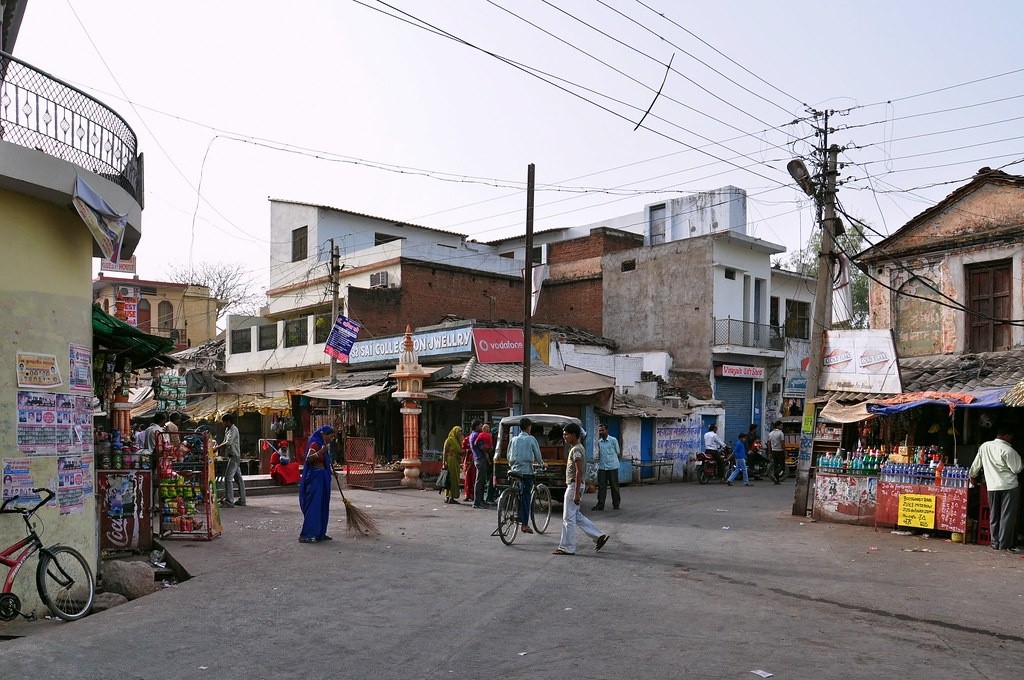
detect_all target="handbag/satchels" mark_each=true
[435,468,451,489]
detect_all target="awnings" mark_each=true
[92,304,180,373]
[299,387,387,400]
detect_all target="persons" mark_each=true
[969,424,1023,550]
[592,425,621,510]
[442,426,462,504]
[507,418,548,534]
[269,441,300,486]
[768,421,786,484]
[299,426,339,543]
[97,426,108,441]
[201,414,247,507]
[744,424,764,481]
[462,421,498,508]
[704,426,728,484]
[553,423,610,555]
[727,433,753,486]
[131,412,180,513]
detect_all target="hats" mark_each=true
[278,440,288,448]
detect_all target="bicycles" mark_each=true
[490,464,552,546]
[0,487,96,623]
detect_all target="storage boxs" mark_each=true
[976,483,991,546]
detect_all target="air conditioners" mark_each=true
[119,286,135,297]
[370,271,388,288]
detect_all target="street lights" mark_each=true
[784,158,838,518]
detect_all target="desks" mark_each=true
[215,460,259,476]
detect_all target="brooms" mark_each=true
[320,430,381,538]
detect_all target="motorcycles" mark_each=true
[693,435,790,485]
[491,413,588,503]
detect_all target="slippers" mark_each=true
[594,536,610,551]
[552,550,576,555]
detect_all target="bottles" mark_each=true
[101,439,149,469]
[817,444,974,488]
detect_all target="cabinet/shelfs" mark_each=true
[95,468,153,552]
[154,430,223,540]
[460,409,488,434]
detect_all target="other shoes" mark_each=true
[444,497,449,502]
[486,499,497,505]
[745,484,753,486]
[727,481,733,486]
[234,501,246,506]
[448,499,461,504]
[219,504,234,507]
[299,536,319,542]
[774,482,781,485]
[318,534,332,540]
[472,501,491,508]
[591,506,599,511]
[614,506,619,509]
[522,526,533,533]
[771,473,778,483]
